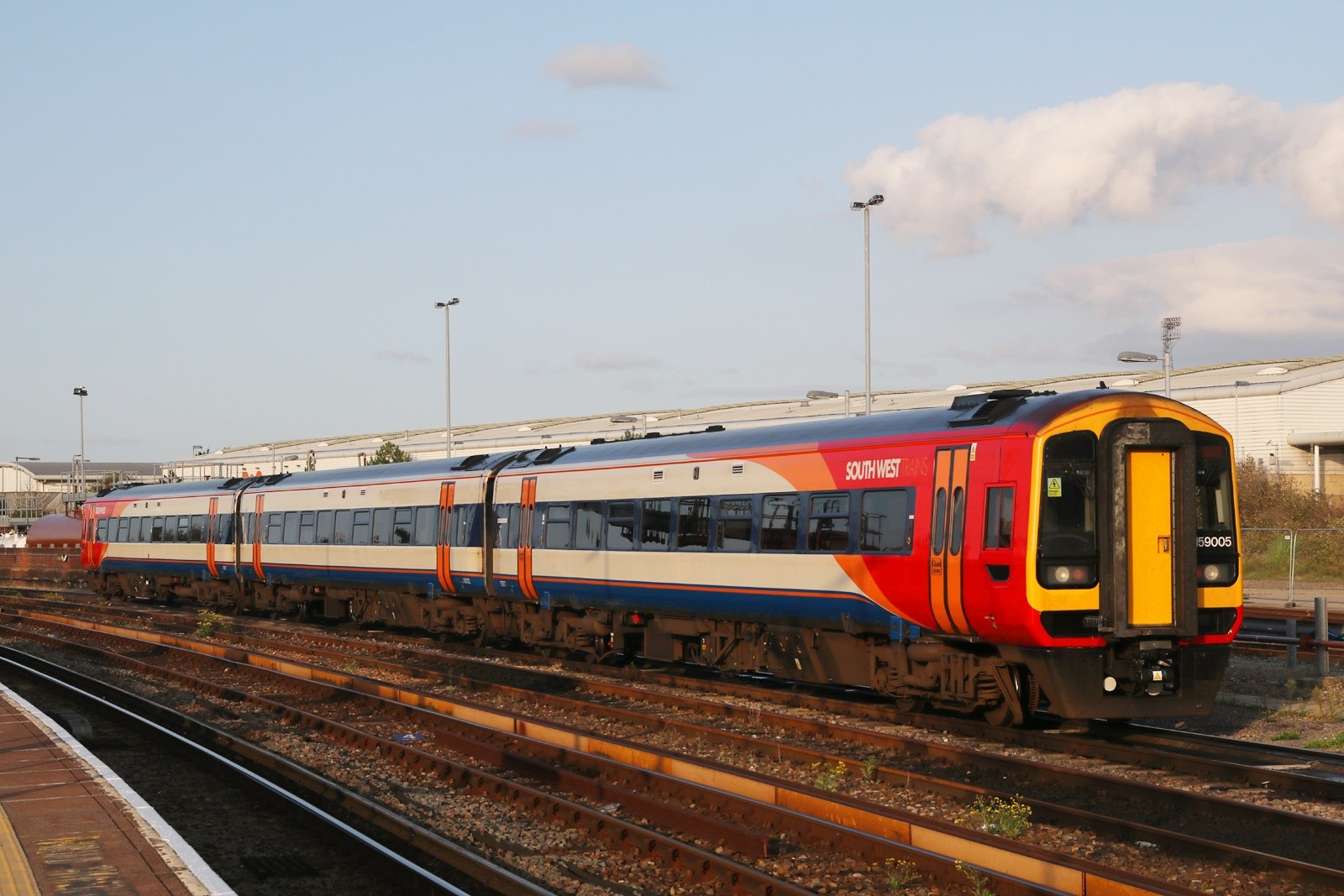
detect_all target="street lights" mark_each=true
[433,298,461,456]
[1118,351,1173,400]
[73,388,89,493]
[850,194,887,416]
[71,454,91,494]
[15,456,41,492]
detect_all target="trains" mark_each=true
[80,385,1249,730]
